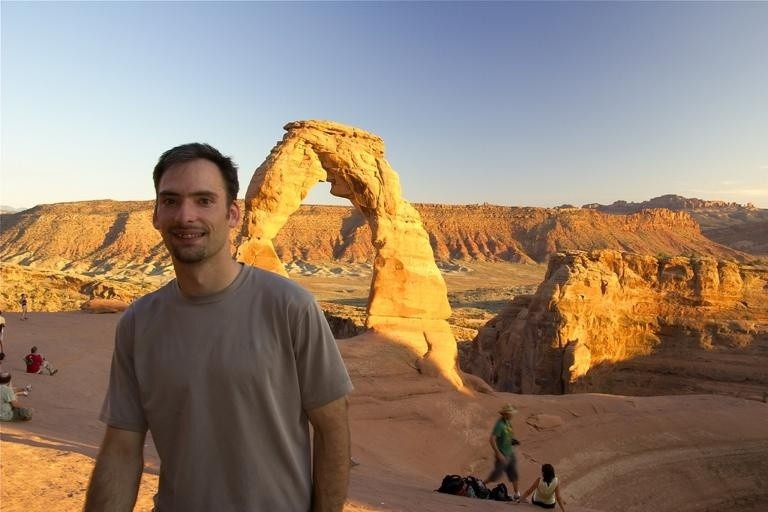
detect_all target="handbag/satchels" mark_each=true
[19,406,33,419]
[439,474,508,502]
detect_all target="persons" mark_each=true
[0,309,9,362]
[21,345,59,376]
[13,384,32,396]
[514,461,567,512]
[481,401,523,500]
[0,371,35,423]
[77,138,355,512]
[19,293,31,321]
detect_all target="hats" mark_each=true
[498,404,518,416]
